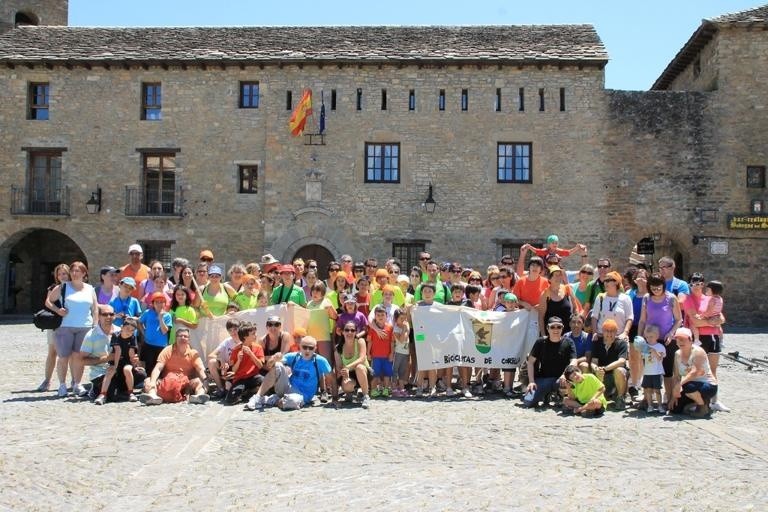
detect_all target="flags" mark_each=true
[289,88,312,137]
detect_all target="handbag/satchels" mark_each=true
[583,293,603,334]
[33,283,65,330]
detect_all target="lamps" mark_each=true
[425,182,436,214]
[86,184,101,214]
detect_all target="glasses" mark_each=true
[268,323,281,327]
[302,345,314,350]
[100,312,114,317]
[344,329,357,332]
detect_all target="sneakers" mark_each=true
[689,403,712,419]
[543,392,551,405]
[563,407,577,416]
[370,372,523,398]
[189,394,210,403]
[128,392,138,402]
[710,401,731,412]
[73,383,88,396]
[37,378,49,392]
[94,392,107,405]
[210,387,280,410]
[58,383,68,397]
[311,391,371,409]
[613,394,668,414]
[581,410,598,418]
[139,393,163,406]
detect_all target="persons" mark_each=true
[37,234,726,418]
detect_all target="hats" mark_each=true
[266,316,281,324]
[547,316,564,328]
[343,295,358,303]
[672,327,693,340]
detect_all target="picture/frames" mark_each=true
[751,199,762,215]
[747,166,765,188]
